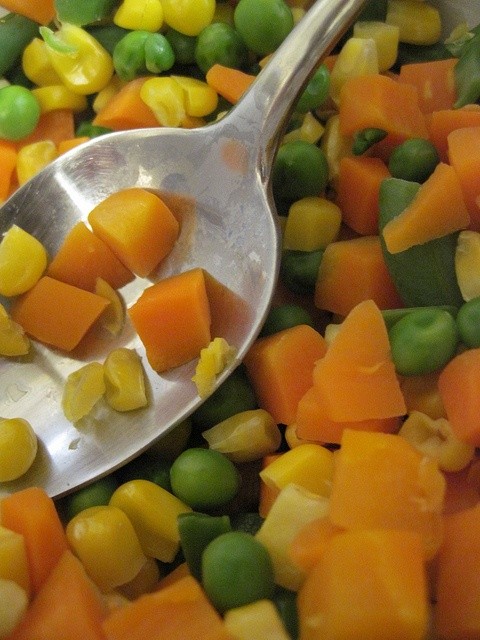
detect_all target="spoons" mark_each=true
[0,0,365,500]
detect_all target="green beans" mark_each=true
[0,0,480,638]
